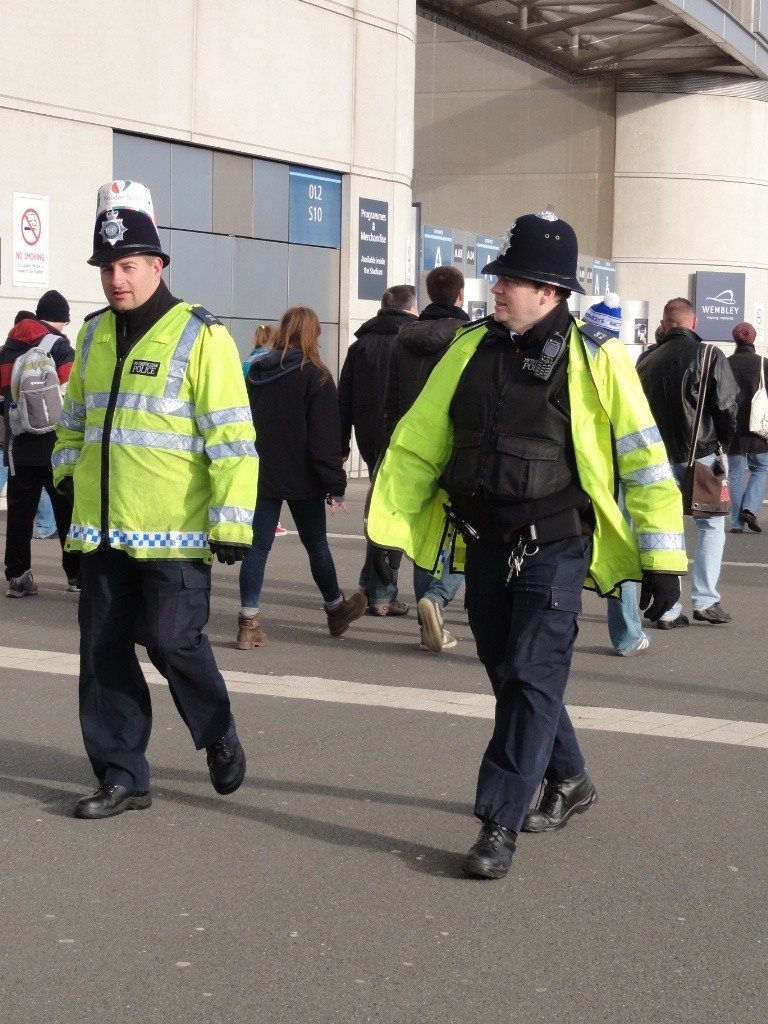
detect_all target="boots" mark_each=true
[234,613,269,650]
[324,589,368,636]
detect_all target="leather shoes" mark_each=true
[521,768,598,833]
[205,711,246,795]
[462,819,518,879]
[75,782,152,819]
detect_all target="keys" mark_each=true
[503,555,524,584]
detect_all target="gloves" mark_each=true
[58,476,74,495]
[210,544,251,565]
[639,572,680,622]
[367,546,403,570]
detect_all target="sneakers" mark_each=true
[65,578,82,592]
[694,602,732,624]
[275,522,287,536]
[616,630,651,657]
[6,569,38,597]
[357,585,457,653]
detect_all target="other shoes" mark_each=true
[740,510,762,532]
[730,529,743,533]
[657,614,688,629]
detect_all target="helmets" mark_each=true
[481,211,586,295]
[87,209,170,269]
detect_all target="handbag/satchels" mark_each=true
[9,335,63,436]
[681,456,733,519]
[749,356,768,438]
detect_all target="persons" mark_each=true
[383,264,471,652]
[50,182,260,819]
[242,325,287,537]
[0,290,82,598]
[726,322,768,533]
[581,292,650,657]
[363,212,688,880]
[635,296,741,631]
[338,284,420,617]
[234,307,368,651]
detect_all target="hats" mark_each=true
[581,292,622,340]
[34,290,69,323]
[732,322,756,345]
